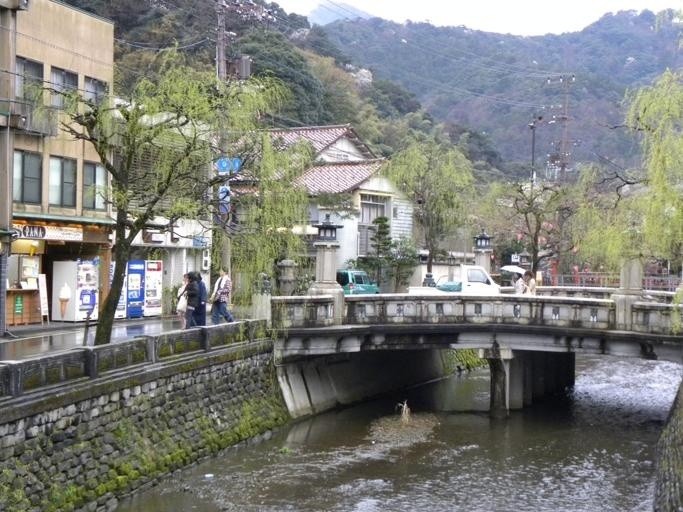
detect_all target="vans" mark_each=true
[337,270,380,295]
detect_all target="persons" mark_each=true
[522,270,536,296]
[209,265,234,324]
[183,272,198,328]
[176,273,189,328]
[192,271,207,326]
[511,273,524,295]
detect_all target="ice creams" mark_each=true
[59,284,71,319]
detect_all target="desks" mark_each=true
[5,289,43,328]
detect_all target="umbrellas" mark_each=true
[499,265,525,275]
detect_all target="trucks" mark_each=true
[408,264,501,296]
